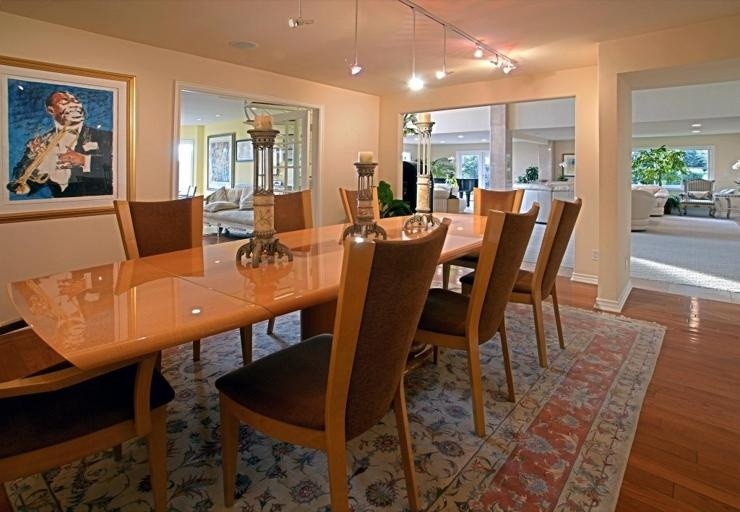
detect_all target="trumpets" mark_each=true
[6,117,80,194]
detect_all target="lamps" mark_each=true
[402,112,442,231]
[236,114,295,268]
[337,150,389,245]
[287,1,519,93]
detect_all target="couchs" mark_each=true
[203,185,255,238]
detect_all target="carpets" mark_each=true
[1,280,670,511]
[523,213,740,293]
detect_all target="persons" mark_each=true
[9,91,112,199]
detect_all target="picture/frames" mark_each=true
[561,152,575,178]
[0,54,140,224]
[238,138,255,163]
[207,132,237,191]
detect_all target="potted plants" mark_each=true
[632,143,689,215]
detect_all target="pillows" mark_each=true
[205,185,255,215]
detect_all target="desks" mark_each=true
[713,192,740,219]
[7,212,489,373]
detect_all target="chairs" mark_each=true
[443,188,525,290]
[113,194,253,366]
[339,188,380,225]
[214,217,452,512]
[255,189,314,334]
[2,315,177,511]
[631,176,718,233]
[405,201,540,436]
[460,196,584,368]
